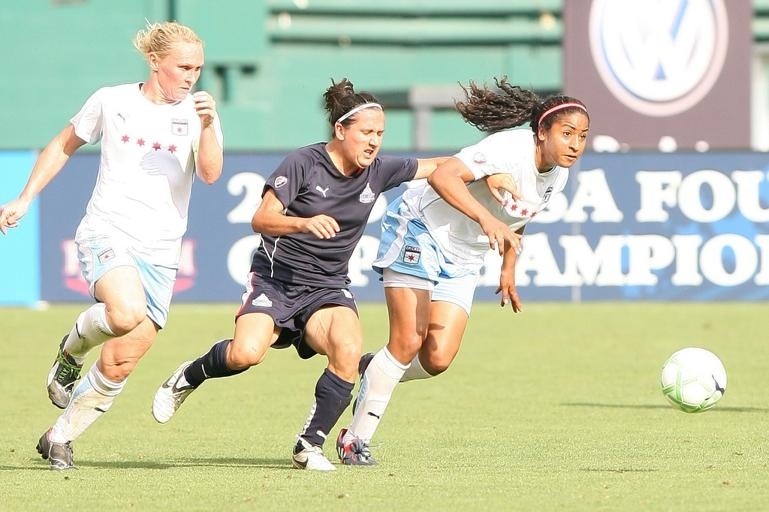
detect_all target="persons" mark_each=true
[334,74,592,466]
[148,75,527,475]
[1,20,227,471]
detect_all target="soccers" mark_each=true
[662,348,727,413]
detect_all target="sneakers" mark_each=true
[34,426,81,471]
[150,358,198,424]
[350,351,378,418]
[290,443,339,471]
[46,332,86,411]
[334,427,380,466]
